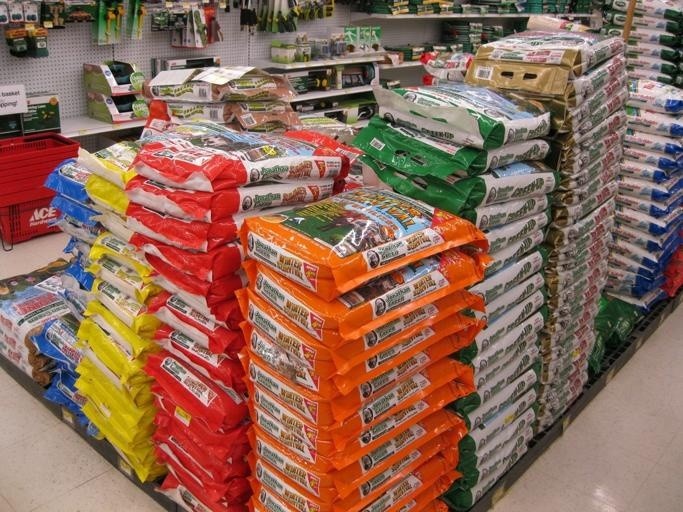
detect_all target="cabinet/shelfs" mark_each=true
[61,1,494,154]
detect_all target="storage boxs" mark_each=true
[0,132,80,251]
